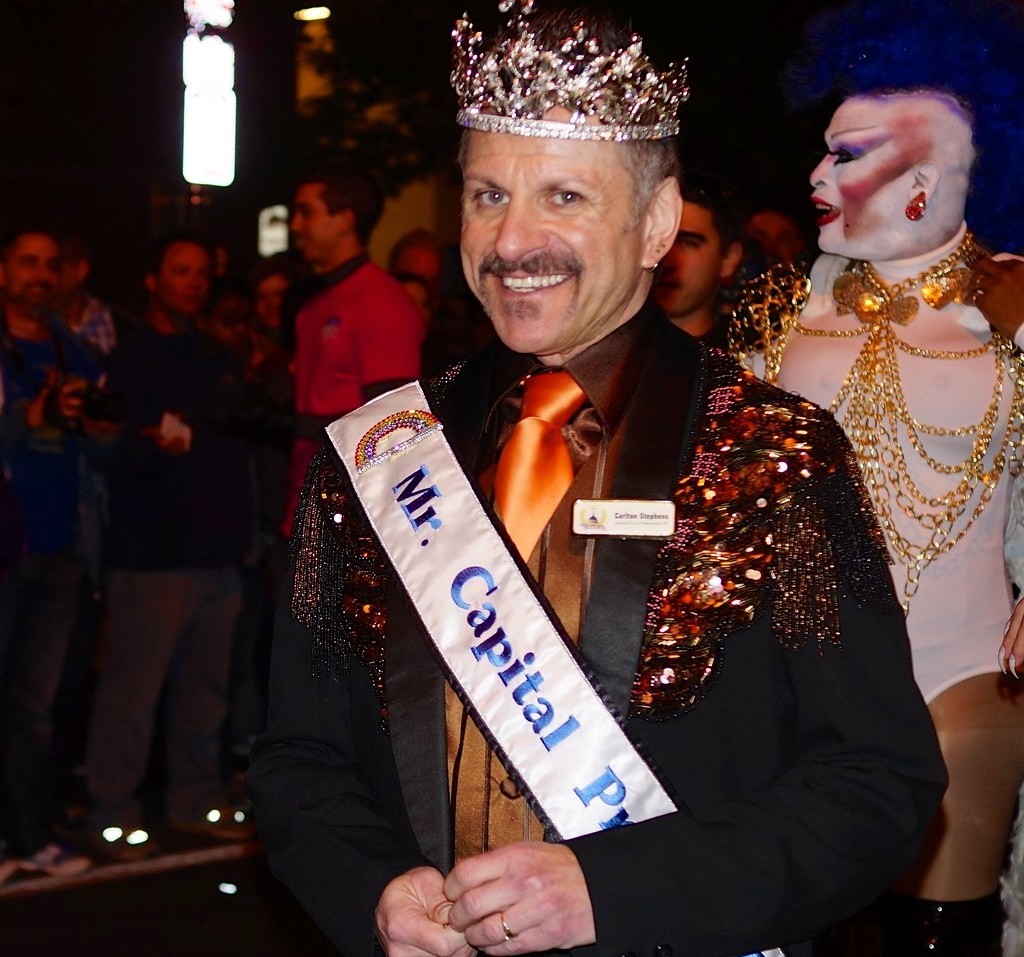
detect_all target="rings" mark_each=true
[500,913,514,942]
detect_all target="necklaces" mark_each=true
[737,233,1024,616]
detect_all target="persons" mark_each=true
[727,0,1024,957]
[656,173,811,361]
[268,165,430,540]
[1,228,521,861]
[288,1,951,957]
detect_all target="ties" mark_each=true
[494,369,585,571]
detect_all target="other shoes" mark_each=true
[171,797,256,839]
[0,857,17,884]
[81,824,158,864]
[13,839,88,877]
